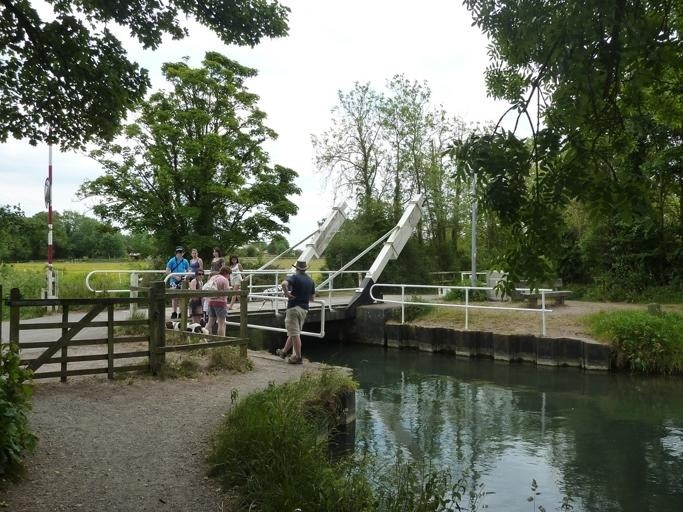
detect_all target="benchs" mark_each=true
[524,290,574,309]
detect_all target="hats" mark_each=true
[291,261,310,271]
[176,246,185,254]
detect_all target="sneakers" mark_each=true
[171,312,177,319]
[287,355,303,364]
[276,349,287,359]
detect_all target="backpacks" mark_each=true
[201,274,223,298]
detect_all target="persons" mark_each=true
[207,248,226,283]
[188,249,205,283]
[228,256,246,310]
[275,261,315,366]
[166,245,190,318]
[206,267,233,336]
[189,270,205,324]
